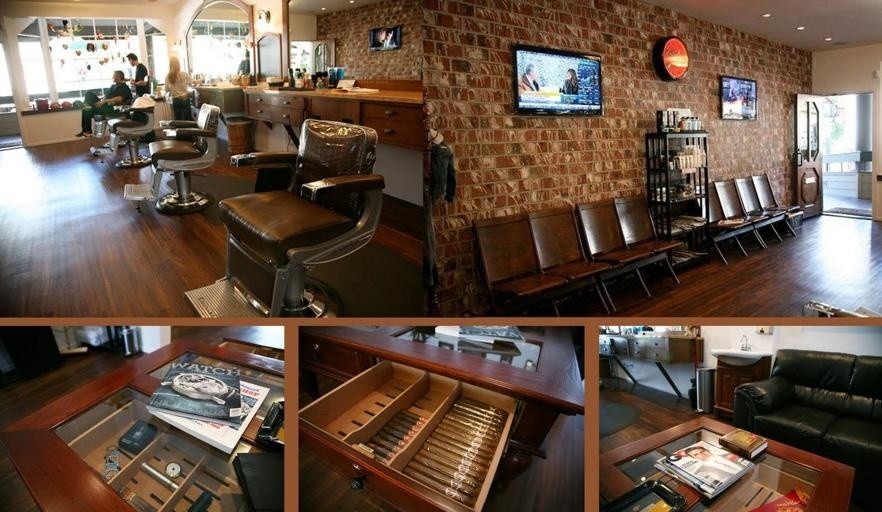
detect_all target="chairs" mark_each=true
[79,81,224,217]
[684,171,806,267]
[465,191,688,317]
[181,114,389,319]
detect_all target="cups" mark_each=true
[328,67,345,88]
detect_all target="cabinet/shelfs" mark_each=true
[197,87,245,113]
[245,88,306,126]
[710,347,774,426]
[310,95,359,130]
[296,325,585,511]
[642,128,714,278]
[627,334,702,366]
[361,99,427,151]
[598,331,630,362]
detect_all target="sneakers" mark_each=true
[74,131,93,138]
[77,102,94,112]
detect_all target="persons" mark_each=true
[383,31,396,49]
[371,30,386,49]
[521,63,541,93]
[558,69,580,98]
[164,57,203,120]
[75,70,134,139]
[237,50,250,76]
[728,86,740,102]
[126,53,153,99]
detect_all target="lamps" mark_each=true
[257,9,270,25]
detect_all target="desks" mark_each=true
[596,416,856,512]
[1,323,287,512]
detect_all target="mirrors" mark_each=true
[279,0,422,82]
[180,0,253,87]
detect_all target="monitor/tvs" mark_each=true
[513,43,604,118]
[368,25,401,51]
[719,73,758,121]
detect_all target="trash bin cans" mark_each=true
[225,116,253,155]
[120,328,139,357]
[695,368,715,414]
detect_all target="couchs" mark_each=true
[731,347,882,512]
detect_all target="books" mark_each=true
[651,437,756,499]
[148,378,271,456]
[717,426,769,459]
[333,84,380,98]
[146,359,245,429]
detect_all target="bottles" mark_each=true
[678,116,706,131]
[670,144,707,168]
[655,186,670,197]
[287,66,328,89]
